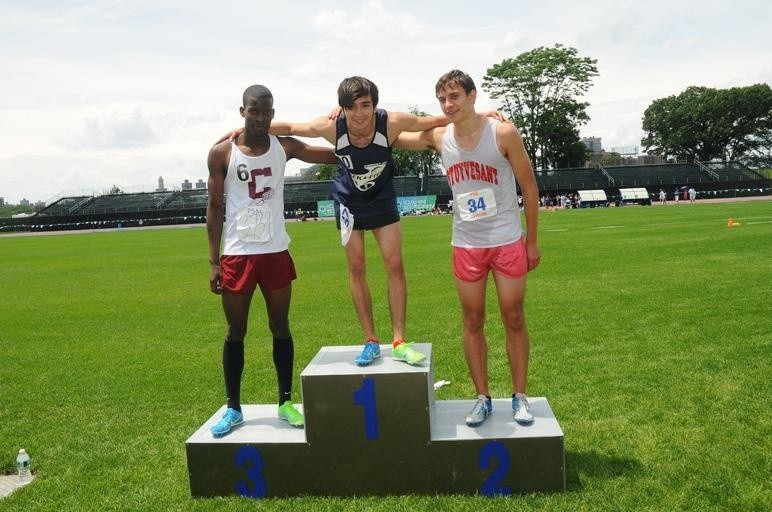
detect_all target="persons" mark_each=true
[400,205,456,216]
[213,77,510,367]
[519,187,698,208]
[392,69,542,426]
[207,83,339,437]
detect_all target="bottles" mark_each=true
[16,446,31,488]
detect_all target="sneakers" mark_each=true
[277,399,305,429]
[391,339,427,366]
[465,393,492,426]
[511,392,534,425]
[354,337,382,366]
[209,407,244,436]
